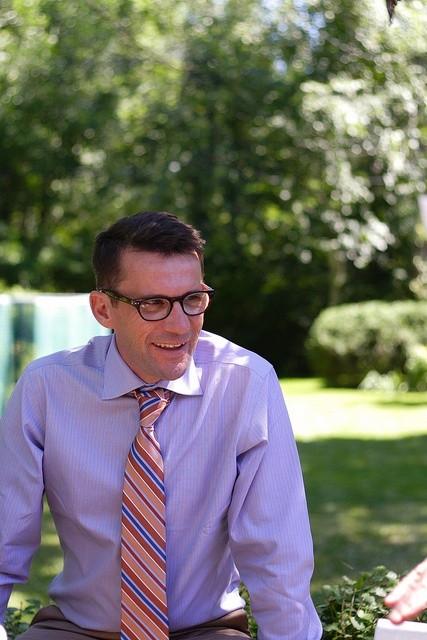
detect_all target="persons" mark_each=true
[373,557,426,636]
[0,209,322,640]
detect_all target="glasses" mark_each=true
[94,281,217,321]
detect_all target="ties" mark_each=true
[120,387,176,640]
